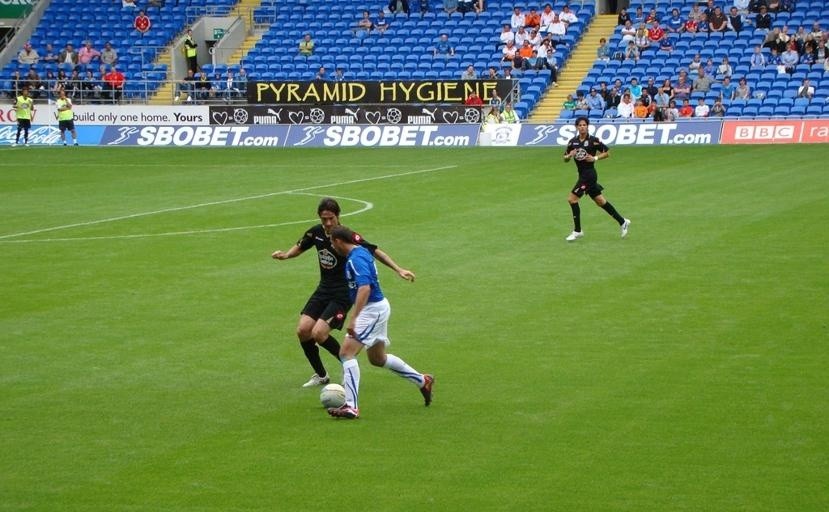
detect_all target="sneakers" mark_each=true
[11,142,79,149]
[327,402,360,420]
[566,230,584,241]
[419,373,436,407]
[619,218,631,239]
[302,371,330,388]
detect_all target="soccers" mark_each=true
[320,383,346,407]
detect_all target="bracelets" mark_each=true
[593,156,599,161]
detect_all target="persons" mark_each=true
[372,11,389,34]
[316,67,332,82]
[433,34,455,57]
[148,0,166,8]
[122,0,137,8]
[563,116,632,242]
[388,1,488,17]
[13,87,36,146]
[488,0,828,121]
[333,68,345,81]
[272,196,417,389]
[299,34,315,56]
[352,11,374,37]
[17,40,126,105]
[327,224,434,419]
[56,90,79,147]
[460,65,477,80]
[181,67,249,98]
[134,10,151,33]
[183,29,198,68]
